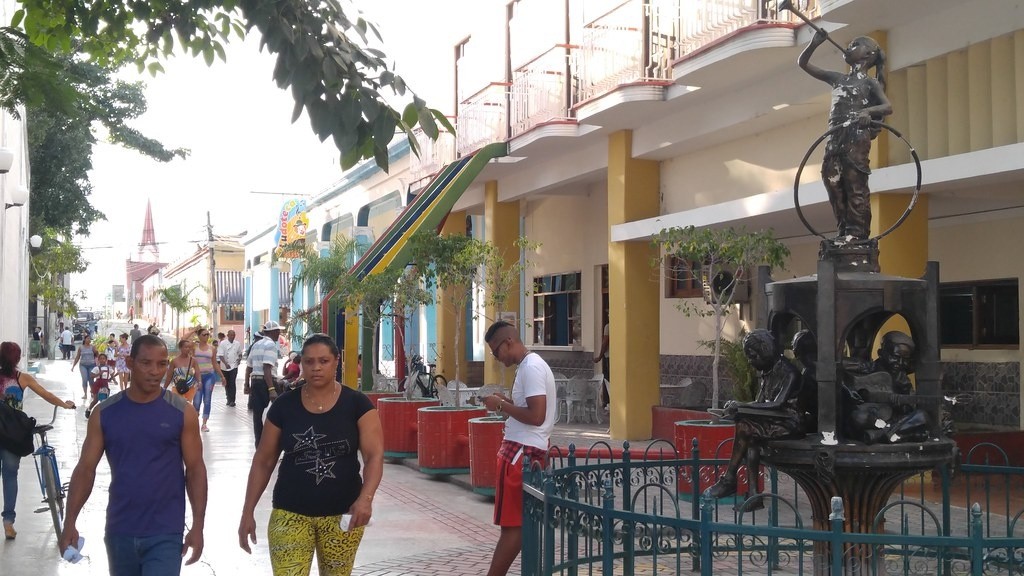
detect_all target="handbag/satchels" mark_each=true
[0,400,36,456]
[175,379,189,394]
[98,386,110,400]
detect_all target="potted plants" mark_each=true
[344,268,441,458]
[337,270,403,407]
[407,230,497,472]
[648,224,792,503]
[455,238,542,498]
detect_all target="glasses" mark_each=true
[492,339,506,358]
[202,333,209,336]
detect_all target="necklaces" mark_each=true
[305,384,336,411]
[510,350,532,395]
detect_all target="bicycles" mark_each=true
[398,353,448,399]
[30,404,76,557]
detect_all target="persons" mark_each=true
[57,335,209,576]
[357,354,362,387]
[0,341,76,539]
[479,321,556,576]
[165,319,303,451]
[71,323,160,419]
[55,326,88,359]
[128,305,134,324]
[238,333,384,576]
[33,327,44,357]
[594,313,609,410]
[797,28,894,246]
[703,329,962,513]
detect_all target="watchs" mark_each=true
[496,399,505,412]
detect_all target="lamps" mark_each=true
[27,234,43,250]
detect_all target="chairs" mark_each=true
[552,373,604,424]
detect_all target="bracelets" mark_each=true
[268,387,276,391]
[361,495,374,502]
[198,382,202,384]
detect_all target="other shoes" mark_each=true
[85,407,91,419]
[82,395,87,399]
[5,524,17,538]
[226,400,236,407]
[201,424,209,432]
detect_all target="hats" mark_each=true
[254,331,264,338]
[263,321,286,332]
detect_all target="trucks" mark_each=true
[73,310,99,339]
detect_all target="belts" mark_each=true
[251,375,277,381]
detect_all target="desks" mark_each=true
[555,379,600,424]
